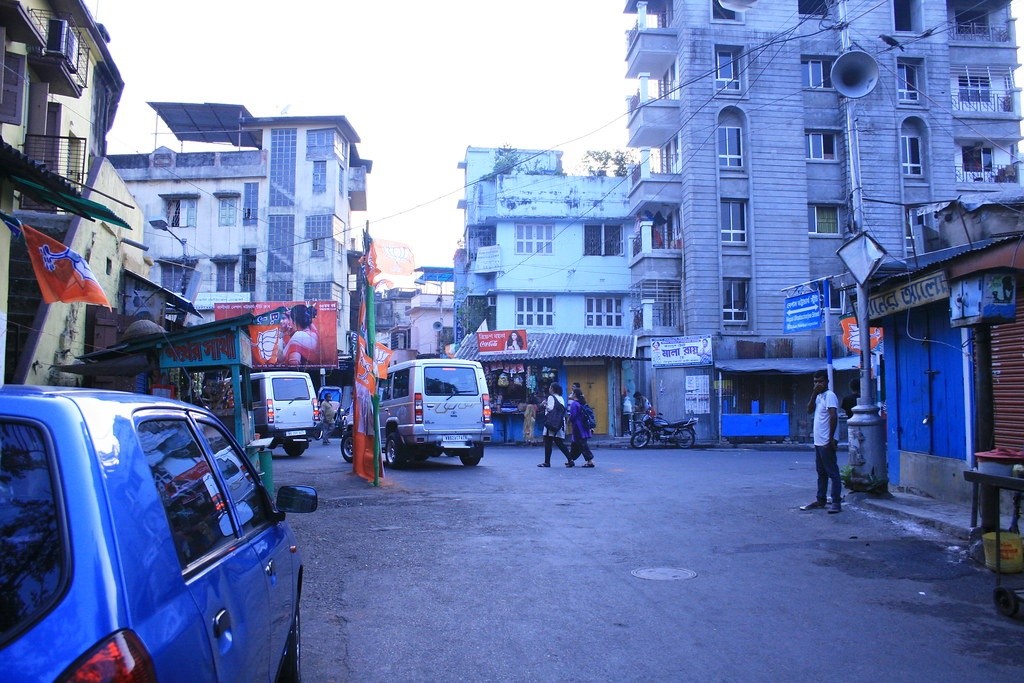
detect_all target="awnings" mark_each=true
[414,266,455,282]
[1,140,133,230]
[147,100,261,148]
[129,269,204,319]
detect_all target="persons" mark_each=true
[321,392,334,444]
[800,368,841,513]
[537,382,595,468]
[504,331,523,351]
[652,342,661,352]
[633,391,652,430]
[840,378,887,421]
[697,339,712,364]
[273,304,324,363]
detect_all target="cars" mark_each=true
[0,381,320,683]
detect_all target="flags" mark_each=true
[19,221,113,313]
[840,316,884,352]
[351,242,393,484]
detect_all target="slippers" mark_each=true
[582,464,595,467]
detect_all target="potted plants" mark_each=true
[612,148,633,176]
[493,142,520,175]
[1004,165,1016,183]
[584,150,612,175]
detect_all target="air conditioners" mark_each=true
[46,18,80,74]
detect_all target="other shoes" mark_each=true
[566,461,574,468]
[537,463,550,467]
[828,503,841,512]
[565,462,575,466]
[806,502,824,508]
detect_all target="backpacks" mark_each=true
[578,402,596,429]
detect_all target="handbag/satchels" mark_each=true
[544,395,566,431]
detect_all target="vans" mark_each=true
[223,371,325,457]
[341,357,495,470]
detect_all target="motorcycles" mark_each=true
[630,402,699,449]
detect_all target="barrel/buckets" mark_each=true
[982,532,1024,574]
